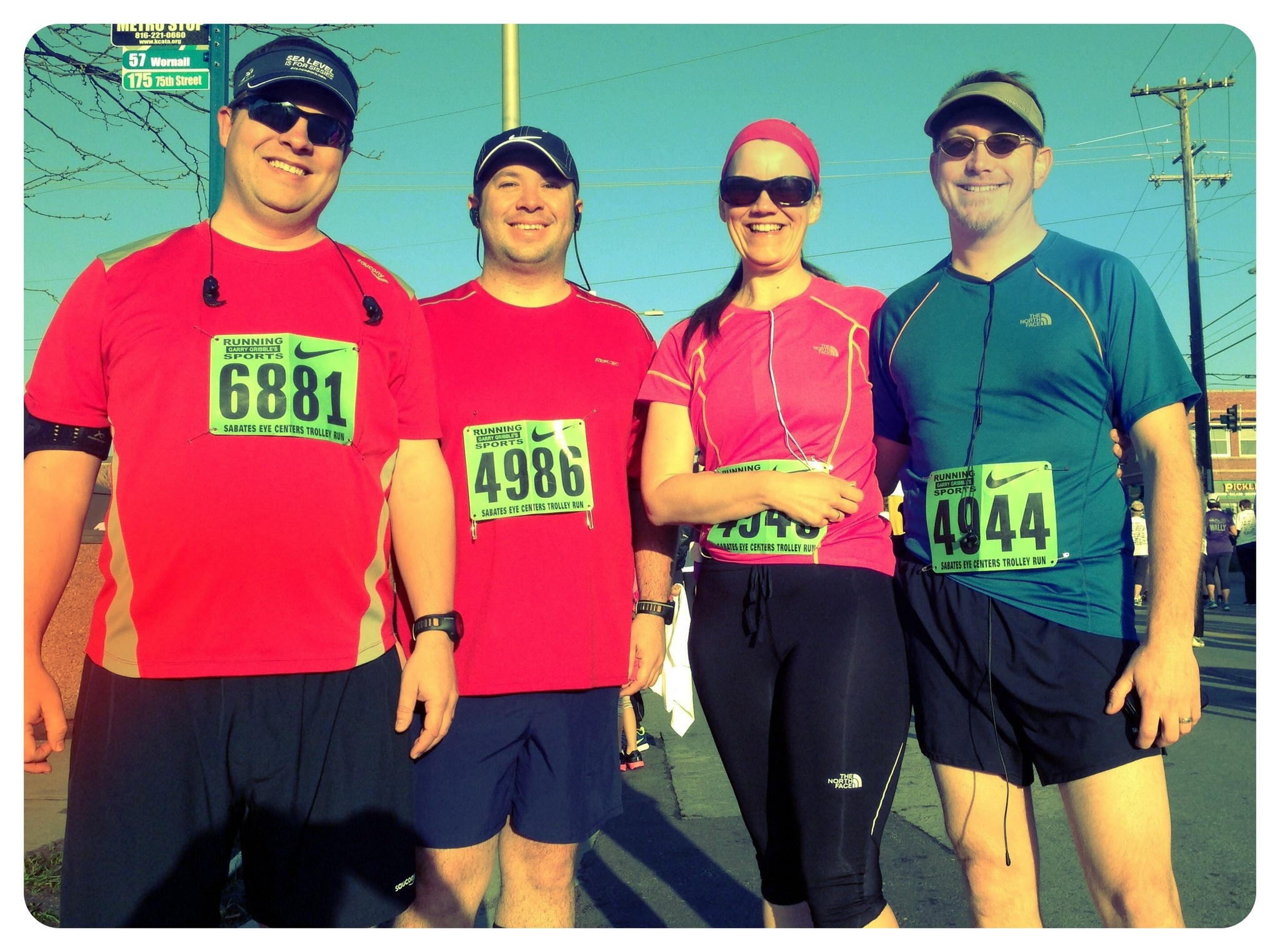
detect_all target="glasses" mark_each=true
[935,131,1044,157]
[719,176,815,208]
[240,98,354,149]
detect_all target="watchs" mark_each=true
[636,597,674,625]
[413,609,464,642]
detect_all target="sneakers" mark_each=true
[620,726,650,754]
[620,750,644,771]
[1222,603,1231,610]
[1205,601,1217,609]
[1192,636,1205,647]
[1135,597,1143,605]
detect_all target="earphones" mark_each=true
[202,275,226,307]
[969,535,979,545]
[362,295,385,324]
[960,533,971,546]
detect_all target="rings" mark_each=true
[829,511,840,522]
[1180,718,1192,723]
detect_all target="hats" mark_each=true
[1208,493,1221,503]
[473,126,580,194]
[925,83,1044,142]
[230,36,359,117]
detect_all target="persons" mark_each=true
[1204,493,1256,610]
[24,35,457,928]
[1191,539,1205,648]
[1128,500,1149,606]
[870,69,1205,929]
[395,125,681,929]
[637,120,1140,929]
[615,688,649,771]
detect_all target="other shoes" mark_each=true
[1243,599,1256,606]
